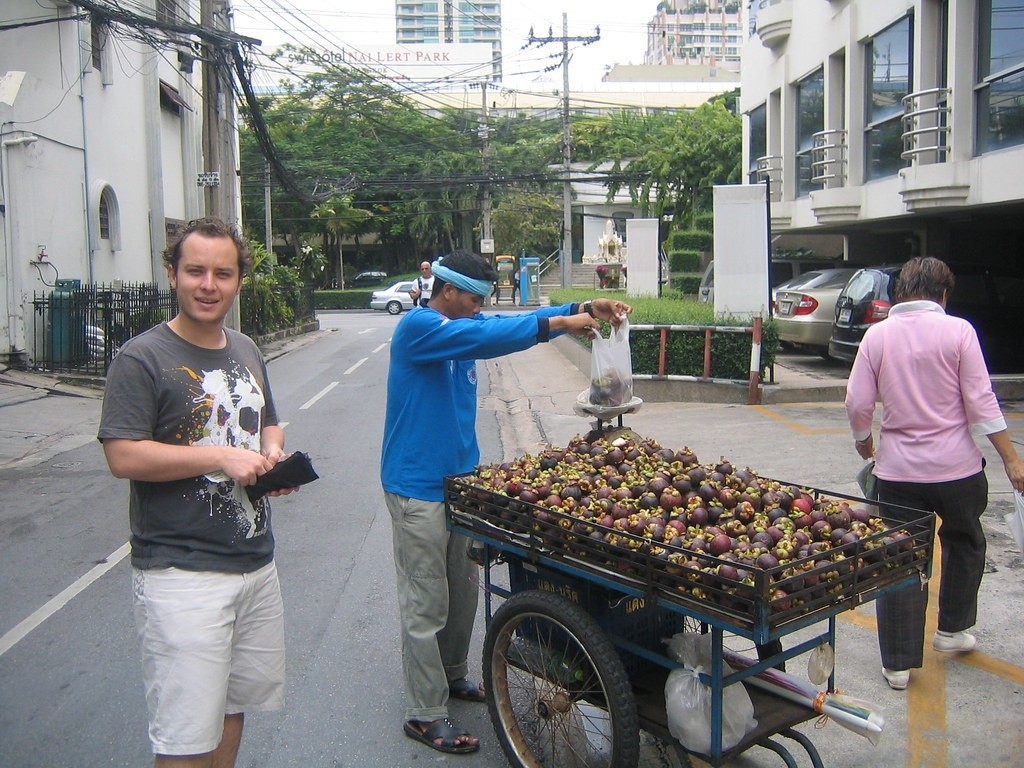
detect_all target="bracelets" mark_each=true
[857,434,871,446]
[583,299,596,321]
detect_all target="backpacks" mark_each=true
[510,268,518,283]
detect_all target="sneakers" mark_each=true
[932,630,976,653]
[882,666,910,689]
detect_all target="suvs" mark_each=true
[698,256,869,304]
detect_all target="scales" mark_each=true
[572,388,643,444]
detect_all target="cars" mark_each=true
[370,280,414,315]
[773,269,860,363]
[772,267,850,315]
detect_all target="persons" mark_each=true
[511,267,521,303]
[376,248,636,756]
[840,253,1023,692]
[408,260,437,307]
[92,219,302,768]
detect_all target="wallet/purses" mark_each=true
[244,450,319,502]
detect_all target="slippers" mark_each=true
[448,678,486,703]
[403,717,480,754]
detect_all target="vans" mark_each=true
[346,270,387,288]
[828,265,901,363]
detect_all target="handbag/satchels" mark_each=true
[1004,489,1024,553]
[589,315,633,406]
[413,277,422,306]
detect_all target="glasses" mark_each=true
[421,268,429,271]
